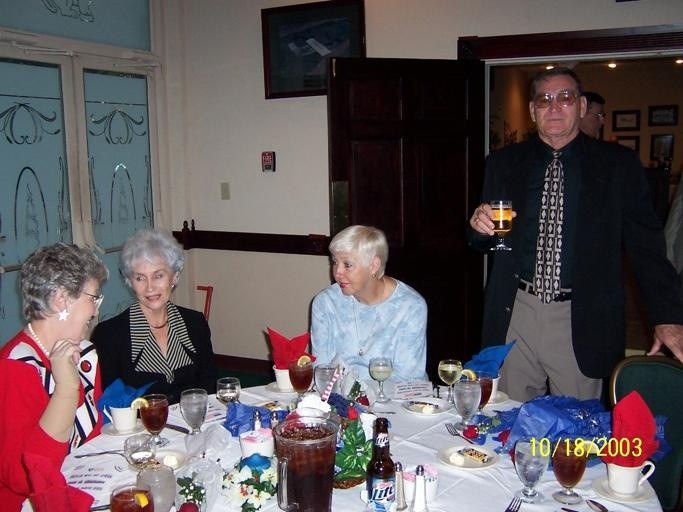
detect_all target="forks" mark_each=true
[503,495,523,512]
[306,383,316,393]
[444,422,474,444]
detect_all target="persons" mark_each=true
[92,227,217,409]
[666,120,683,273]
[0,242,110,512]
[581,91,605,138]
[467,65,682,400]
[307,226,429,392]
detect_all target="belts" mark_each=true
[517,281,574,302]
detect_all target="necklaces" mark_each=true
[149,321,168,330]
[353,282,386,356]
[26,322,50,359]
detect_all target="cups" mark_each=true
[236,429,275,460]
[605,460,655,495]
[103,402,138,432]
[271,416,339,512]
[296,407,329,424]
[272,365,291,392]
[487,373,500,399]
[402,468,439,504]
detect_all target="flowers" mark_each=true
[496,429,512,447]
[461,407,509,442]
[219,466,281,512]
[330,386,378,486]
[171,473,205,512]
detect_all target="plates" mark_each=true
[265,381,297,397]
[401,396,450,416]
[98,417,147,437]
[434,444,498,470]
[591,474,655,506]
[484,389,508,405]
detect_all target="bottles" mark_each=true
[365,417,394,512]
[269,410,278,427]
[392,459,428,511]
[251,409,263,428]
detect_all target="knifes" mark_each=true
[74,449,123,458]
[561,505,579,512]
[586,498,609,512]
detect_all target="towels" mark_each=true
[265,323,319,370]
[464,338,520,382]
[599,387,661,469]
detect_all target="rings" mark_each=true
[475,218,478,223]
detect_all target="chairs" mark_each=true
[607,346,682,512]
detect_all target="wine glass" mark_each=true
[510,435,551,502]
[289,358,342,404]
[110,376,240,512]
[552,440,587,505]
[437,358,462,403]
[367,357,393,403]
[485,199,516,252]
[450,370,492,428]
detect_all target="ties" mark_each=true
[534,149,565,303]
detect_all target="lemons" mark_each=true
[460,369,477,380]
[134,493,149,508]
[131,397,149,410]
[297,355,311,368]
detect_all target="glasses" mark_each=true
[588,111,606,122]
[531,89,579,109]
[80,290,104,310]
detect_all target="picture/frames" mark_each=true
[258,0,369,102]
[608,100,678,166]
